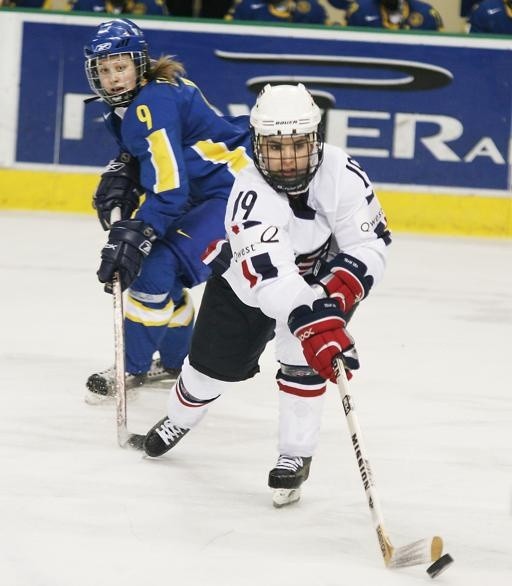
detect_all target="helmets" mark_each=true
[83,17,150,106]
[248,81,324,192]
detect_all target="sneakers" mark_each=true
[142,415,191,457]
[268,455,312,488]
[142,357,178,381]
[86,364,144,395]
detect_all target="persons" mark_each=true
[86,19,254,396]
[144,80,394,489]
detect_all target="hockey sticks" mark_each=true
[331,356,442,567]
[105,159,146,451]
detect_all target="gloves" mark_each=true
[314,252,374,314]
[287,297,360,384]
[96,218,158,294]
[92,158,144,231]
